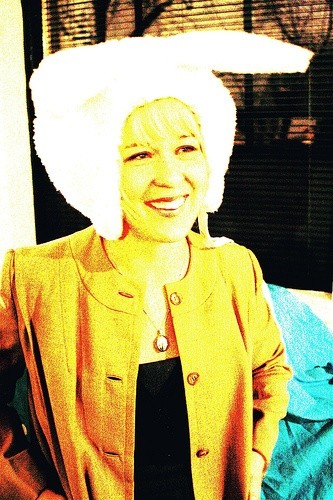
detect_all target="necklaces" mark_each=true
[98,241,187,352]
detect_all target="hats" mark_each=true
[29,29,315,249]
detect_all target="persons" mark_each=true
[0,32,314,500]
